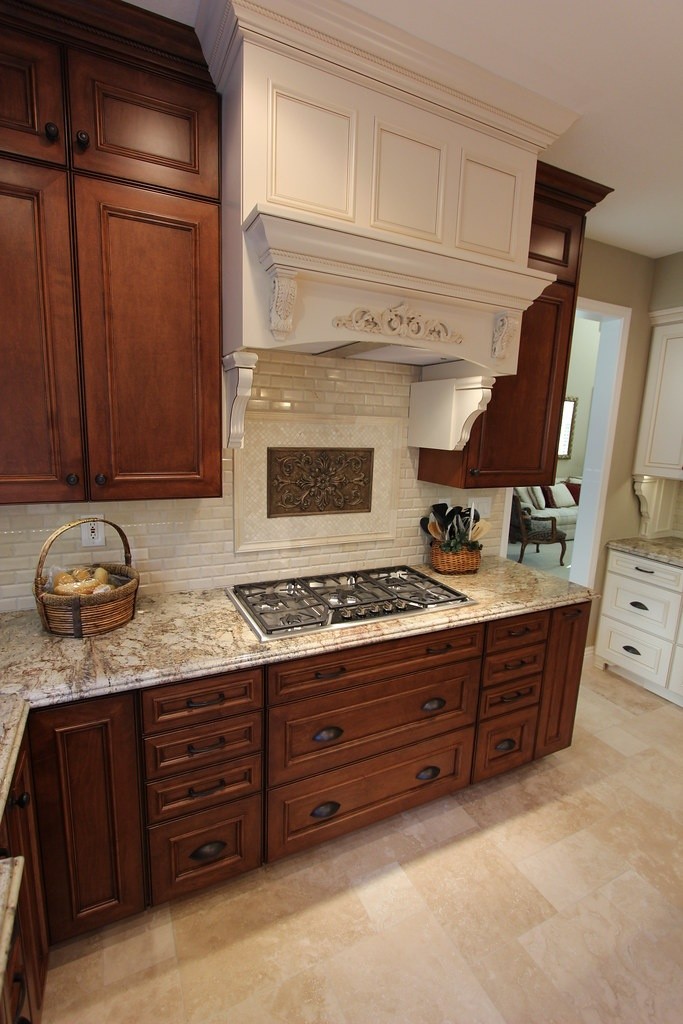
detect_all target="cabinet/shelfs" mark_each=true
[595,548,682,709]
[0,597,595,1024]
[632,320,683,477]
[0,0,225,505]
[417,158,614,489]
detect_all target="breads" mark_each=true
[52,566,114,596]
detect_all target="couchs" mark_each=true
[512,478,583,541]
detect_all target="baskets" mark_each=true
[33,518,139,638]
[429,502,480,575]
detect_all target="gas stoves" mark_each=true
[225,564,477,642]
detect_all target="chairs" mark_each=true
[512,494,567,566]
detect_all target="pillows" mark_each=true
[520,482,580,510]
